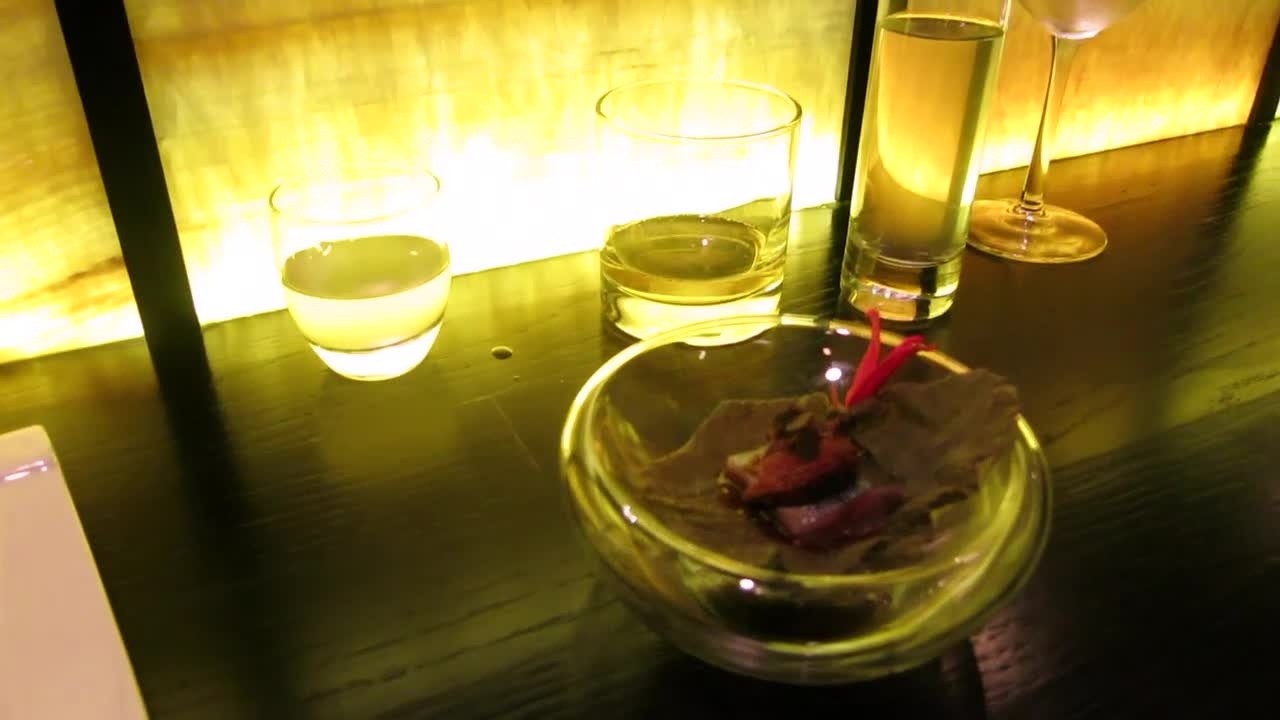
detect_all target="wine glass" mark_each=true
[967,0,1141,265]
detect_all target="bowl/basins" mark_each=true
[560,313,1052,681]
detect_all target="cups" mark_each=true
[270,165,451,383]
[841,0,1010,323]
[594,78,803,345]
